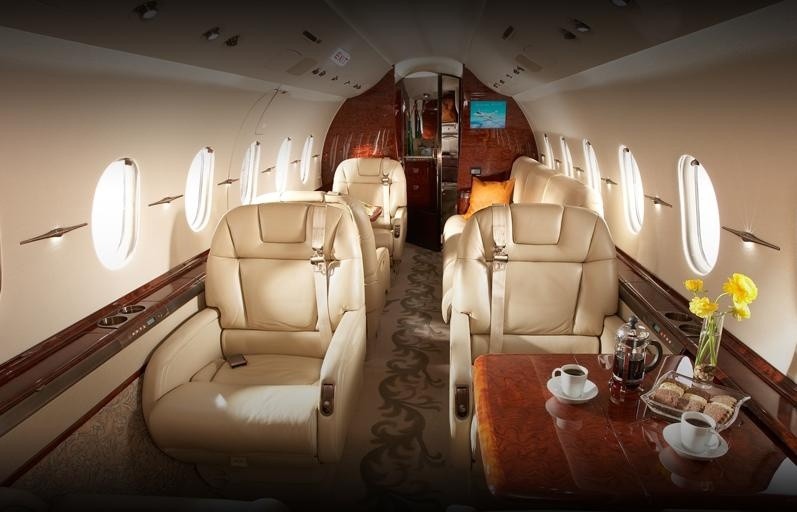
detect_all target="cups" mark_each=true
[551,363,589,398]
[680,411,721,454]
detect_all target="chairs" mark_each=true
[331,157,408,262]
[251,191,391,341]
[447,203,630,479]
[142,203,367,504]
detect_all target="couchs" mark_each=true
[441,156,604,245]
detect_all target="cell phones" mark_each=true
[226,354,247,368]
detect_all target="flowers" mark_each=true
[683,273,758,366]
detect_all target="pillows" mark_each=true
[464,177,516,221]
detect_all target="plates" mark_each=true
[662,423,729,460]
[546,376,599,405]
[639,370,752,433]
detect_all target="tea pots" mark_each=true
[607,315,663,402]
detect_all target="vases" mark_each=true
[692,312,724,389]
